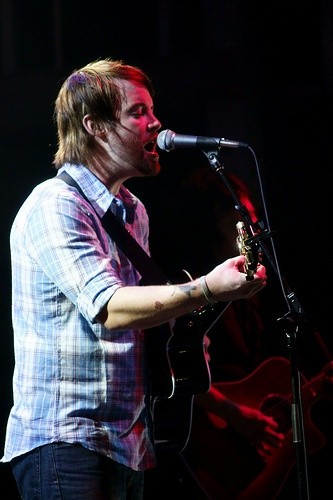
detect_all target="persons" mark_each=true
[0,60,268,500]
[212,208,269,370]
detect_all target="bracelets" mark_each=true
[199,275,218,304]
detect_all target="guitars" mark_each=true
[142,214,269,461]
[192,355,333,478]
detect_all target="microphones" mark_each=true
[156,129,246,152]
